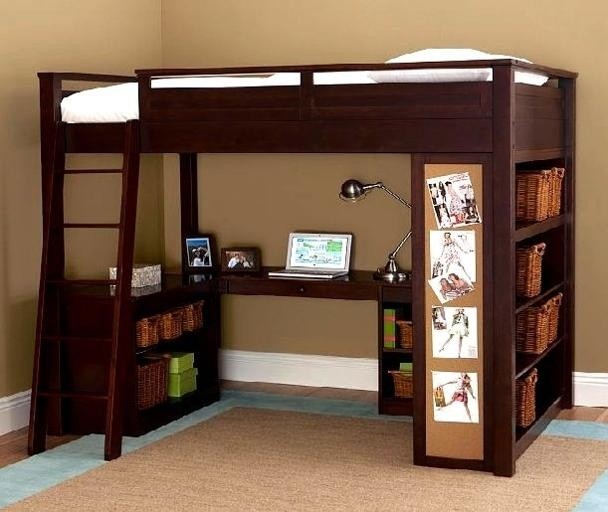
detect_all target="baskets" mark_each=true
[516,243,546,298]
[136,357,170,410]
[396,321,413,348]
[182,300,204,331]
[540,292,563,343]
[517,167,566,221]
[160,307,184,339]
[516,367,538,428]
[137,314,162,348]
[388,370,413,399]
[516,170,551,222]
[516,299,553,355]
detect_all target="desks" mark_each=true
[164,266,413,417]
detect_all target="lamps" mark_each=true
[339,179,412,281]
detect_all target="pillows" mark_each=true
[368,48,550,87]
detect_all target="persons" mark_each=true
[444,181,465,224]
[227,252,240,270]
[241,256,252,269]
[439,277,461,302]
[436,232,473,281]
[448,273,474,296]
[437,307,470,358]
[190,246,209,266]
[436,372,477,420]
[437,181,452,222]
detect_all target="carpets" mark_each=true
[1,388,607,512]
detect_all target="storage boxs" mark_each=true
[167,368,199,397]
[169,352,194,374]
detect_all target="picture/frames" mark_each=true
[182,233,218,275]
[221,246,262,275]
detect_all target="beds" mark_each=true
[27,58,579,478]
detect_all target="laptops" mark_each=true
[267,232,353,279]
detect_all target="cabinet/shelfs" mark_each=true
[47,273,219,438]
